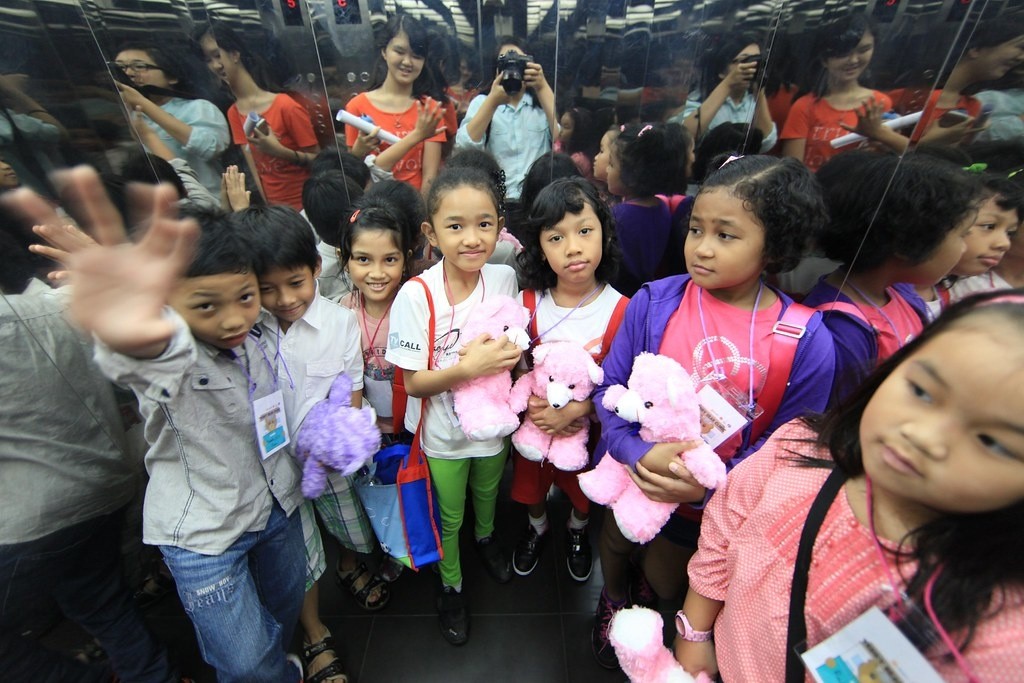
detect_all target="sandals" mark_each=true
[335,563,390,611]
[303,627,348,683]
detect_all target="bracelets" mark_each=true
[304,152,307,164]
[295,151,300,165]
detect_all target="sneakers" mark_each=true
[439,585,468,644]
[592,585,631,668]
[512,516,553,576]
[636,576,658,609]
[475,534,514,584]
[565,518,593,581]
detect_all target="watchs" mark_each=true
[675,610,711,642]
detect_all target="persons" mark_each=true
[227,204,385,610]
[674,288,1024,683]
[339,206,413,586]
[384,166,530,648]
[12,164,308,682]
[512,175,632,582]
[299,95,448,303]
[594,155,836,667]
[0,14,1024,683]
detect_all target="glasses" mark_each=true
[116,62,175,77]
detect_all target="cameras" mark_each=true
[746,54,768,93]
[498,49,535,95]
[109,63,145,98]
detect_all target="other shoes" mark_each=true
[179,677,196,683]
[286,654,303,683]
[380,555,404,582]
[132,571,172,607]
[75,638,107,663]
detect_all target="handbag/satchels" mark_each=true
[312,470,372,553]
[355,445,444,571]
